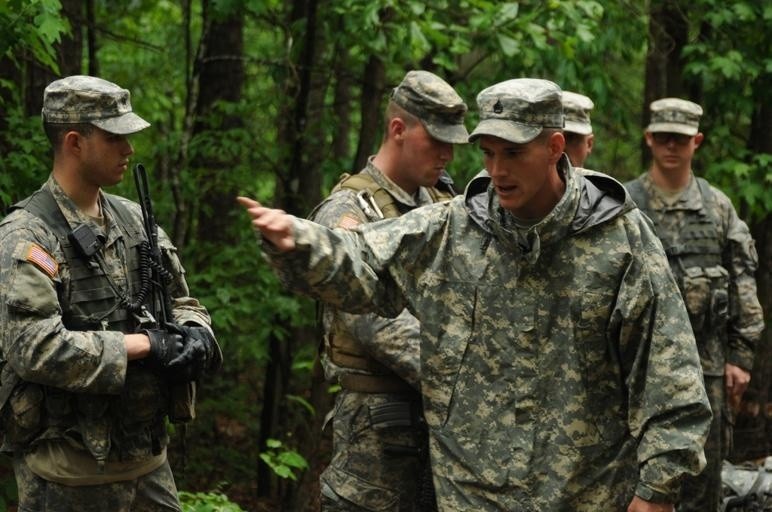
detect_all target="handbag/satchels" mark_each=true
[684,264,730,323]
[0,368,170,466]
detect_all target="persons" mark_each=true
[236,77,714,512]
[0,75,224,512]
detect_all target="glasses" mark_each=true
[653,133,691,146]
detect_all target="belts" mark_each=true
[337,374,407,393]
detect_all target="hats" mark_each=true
[647,97,703,136]
[468,78,595,144]
[42,75,151,135]
[390,70,473,144]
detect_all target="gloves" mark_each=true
[143,322,218,368]
